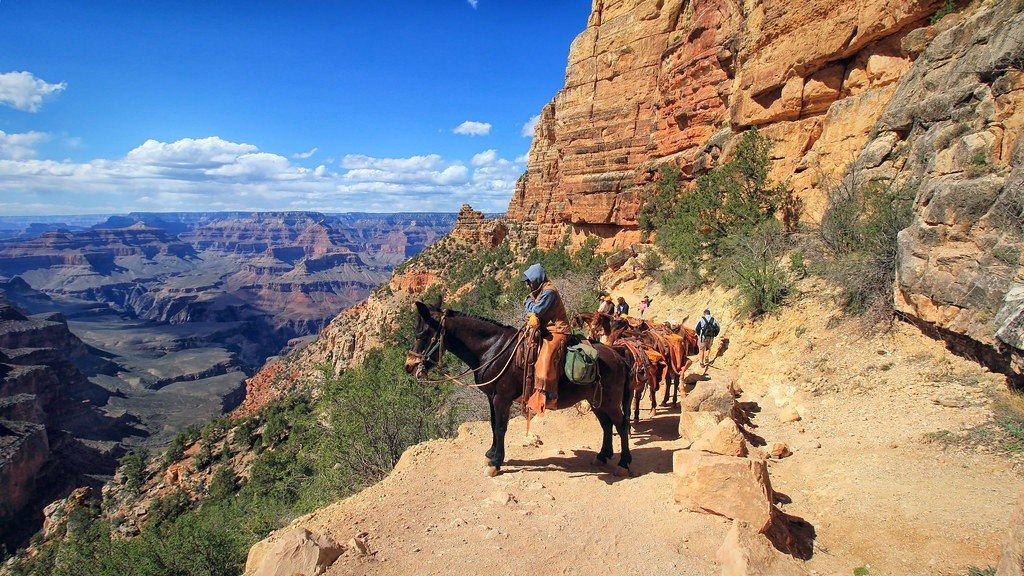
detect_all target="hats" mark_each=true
[520,274,528,282]
[600,295,605,300]
[604,296,612,302]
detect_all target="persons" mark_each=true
[598,296,615,317]
[512,263,572,423]
[614,297,629,317]
[695,309,720,368]
[637,301,647,316]
[643,295,654,307]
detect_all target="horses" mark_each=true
[401,289,699,481]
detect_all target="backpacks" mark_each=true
[702,317,714,337]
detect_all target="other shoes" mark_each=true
[705,356,709,363]
[700,363,705,368]
[525,397,558,411]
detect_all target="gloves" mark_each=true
[529,313,540,328]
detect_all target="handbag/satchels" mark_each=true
[565,344,599,385]
